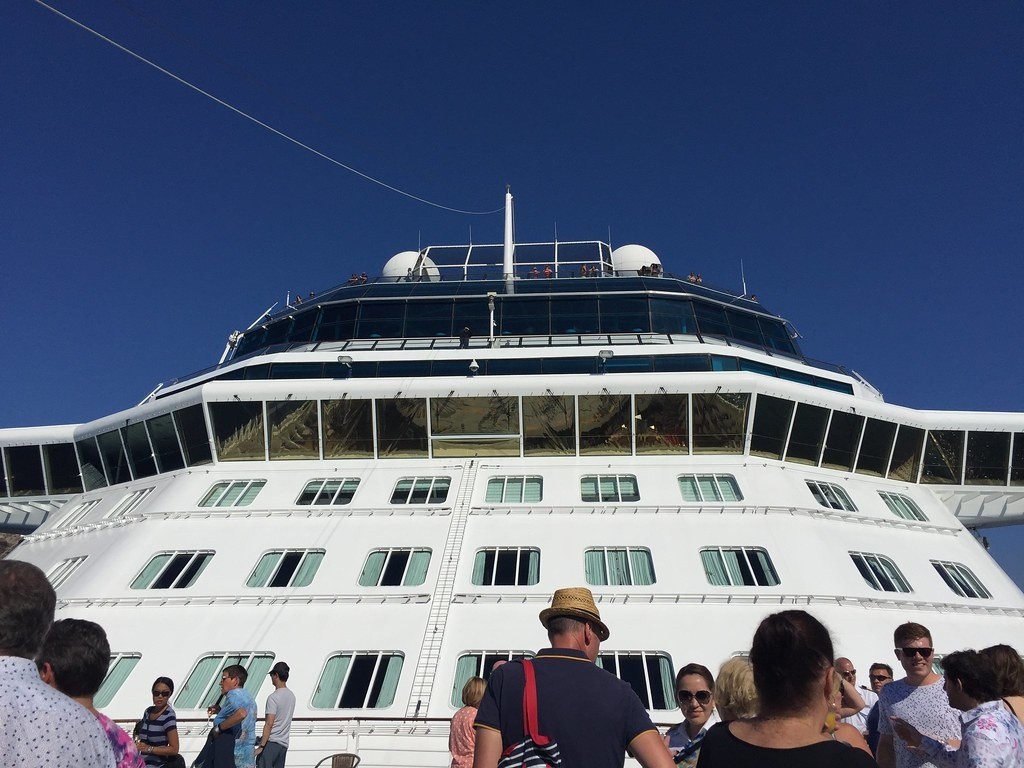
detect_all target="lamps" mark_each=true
[469,359,479,372]
[600,350,613,373]
[338,356,352,378]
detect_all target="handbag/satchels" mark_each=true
[497,660,562,768]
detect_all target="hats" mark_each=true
[539,587,610,642]
[267,662,289,674]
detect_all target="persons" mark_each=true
[581,264,599,277]
[135,677,179,768]
[640,264,660,276]
[459,327,472,349]
[405,268,412,281]
[668,610,1024,768]
[752,295,756,301]
[689,272,702,284]
[449,586,676,768]
[531,266,552,278]
[0,559,116,768]
[293,292,314,305]
[35,618,147,768]
[212,661,296,768]
[349,272,367,285]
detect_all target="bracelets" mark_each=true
[218,724,222,732]
[148,746,153,753]
[260,745,264,748]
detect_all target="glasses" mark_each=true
[843,669,856,677]
[678,690,713,704]
[153,691,169,697]
[870,675,893,681]
[839,683,844,696]
[896,648,933,658]
[222,675,236,682]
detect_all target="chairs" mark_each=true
[314,754,361,768]
